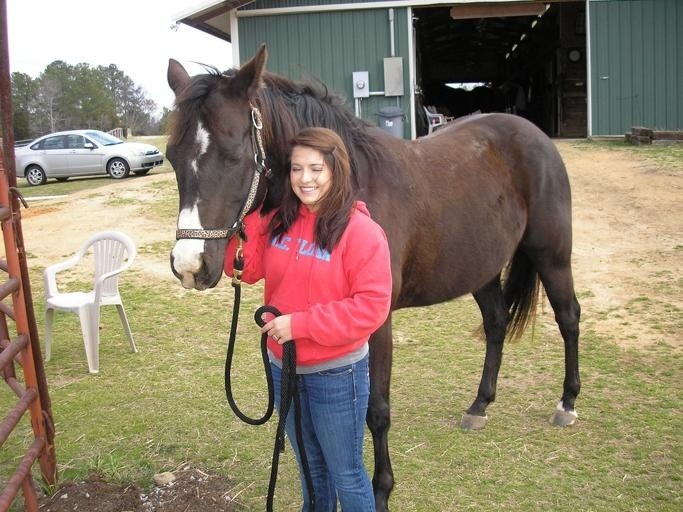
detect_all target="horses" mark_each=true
[163,40,582,512]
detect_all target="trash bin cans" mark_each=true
[373,107,408,140]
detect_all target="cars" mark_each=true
[14,129,165,186]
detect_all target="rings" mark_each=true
[273,335,279,341]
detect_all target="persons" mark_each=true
[225,127,394,512]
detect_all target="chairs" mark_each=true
[42,230,139,376]
[421,105,455,134]
